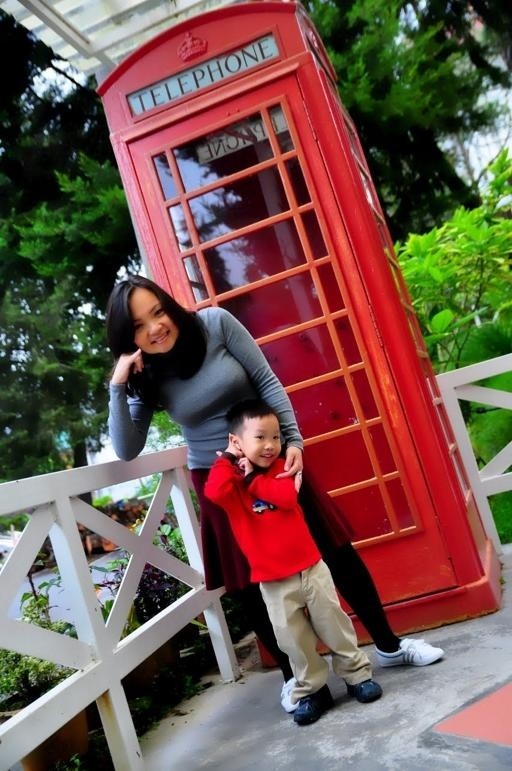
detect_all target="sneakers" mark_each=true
[281,675,301,713]
[374,637,444,667]
[293,684,334,726]
[346,678,382,703]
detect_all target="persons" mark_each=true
[202,398,383,727]
[105,276,445,713]
